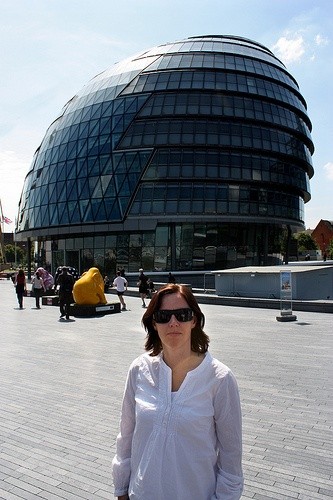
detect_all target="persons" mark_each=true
[114,283,244,500]
[55,267,75,320]
[136,268,148,308]
[104,264,175,299]
[113,270,128,311]
[323,252,328,262]
[10,265,47,309]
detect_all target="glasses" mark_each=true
[153,308,195,323]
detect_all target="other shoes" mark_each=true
[66,314,69,320]
[60,313,66,318]
[20,304,23,308]
[36,305,41,309]
[122,304,126,309]
[142,307,146,308]
[142,305,146,306]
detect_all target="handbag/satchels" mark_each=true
[23,285,27,297]
